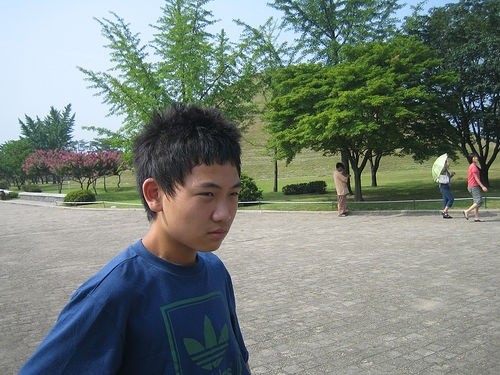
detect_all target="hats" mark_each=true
[336,163,345,169]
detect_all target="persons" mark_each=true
[437,159,456,218]
[463,152,488,222]
[334,162,351,217]
[18,102,250,375]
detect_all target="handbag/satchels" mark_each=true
[436,171,449,184]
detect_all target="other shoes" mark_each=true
[474,218,484,221]
[463,210,469,220]
[338,212,348,216]
[442,211,452,218]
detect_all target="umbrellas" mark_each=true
[432,153,455,182]
